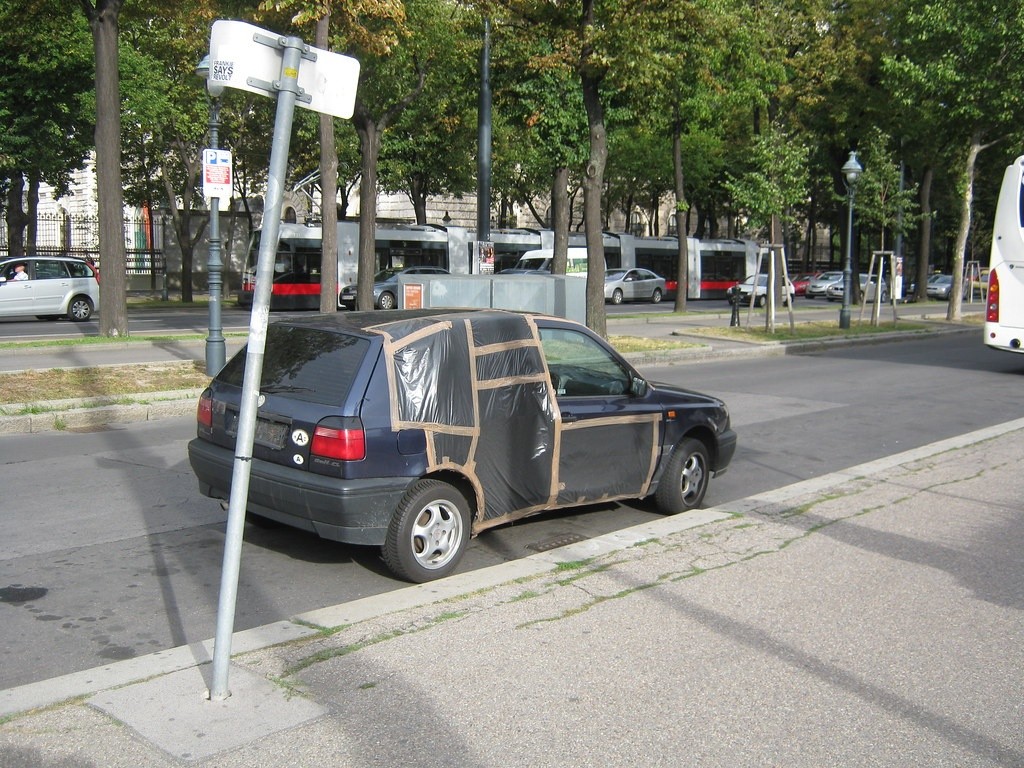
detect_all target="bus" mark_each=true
[984,154,1024,353]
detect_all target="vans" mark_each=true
[514,249,608,281]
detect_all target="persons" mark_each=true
[14,264,28,280]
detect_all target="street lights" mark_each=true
[839,149,863,329]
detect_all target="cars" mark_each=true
[604,267,669,305]
[805,271,843,299]
[790,272,824,297]
[906,266,989,301]
[238,268,321,311]
[727,274,796,308]
[339,265,452,312]
[186,305,739,584]
[0,255,101,322]
[827,273,889,304]
